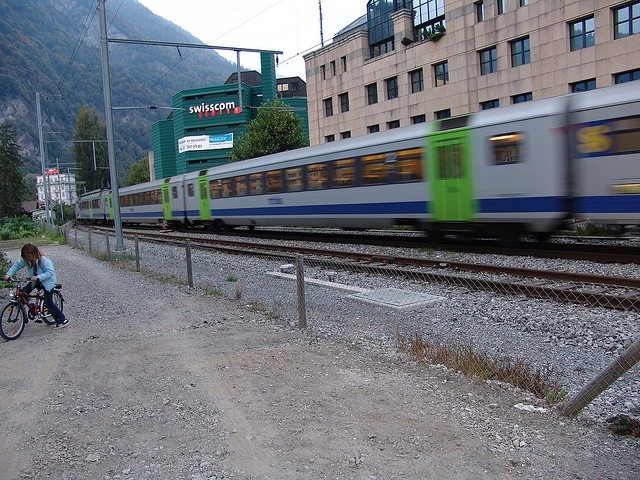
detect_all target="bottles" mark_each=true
[10,288,17,301]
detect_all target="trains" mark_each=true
[75,78,640,249]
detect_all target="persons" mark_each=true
[4,242,70,330]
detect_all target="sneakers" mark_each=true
[53,319,70,329]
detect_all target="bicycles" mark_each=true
[1,275,65,340]
